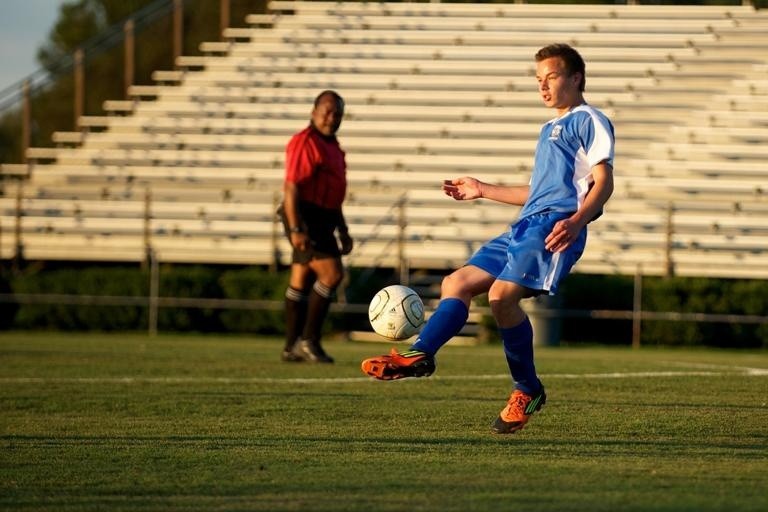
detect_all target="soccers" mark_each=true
[367,284,426,344]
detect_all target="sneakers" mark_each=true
[301,338,333,364]
[280,350,304,362]
[490,380,547,434]
[361,348,436,381]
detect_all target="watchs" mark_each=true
[290,227,304,232]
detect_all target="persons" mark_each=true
[362,43,614,435]
[275,90,353,364]
